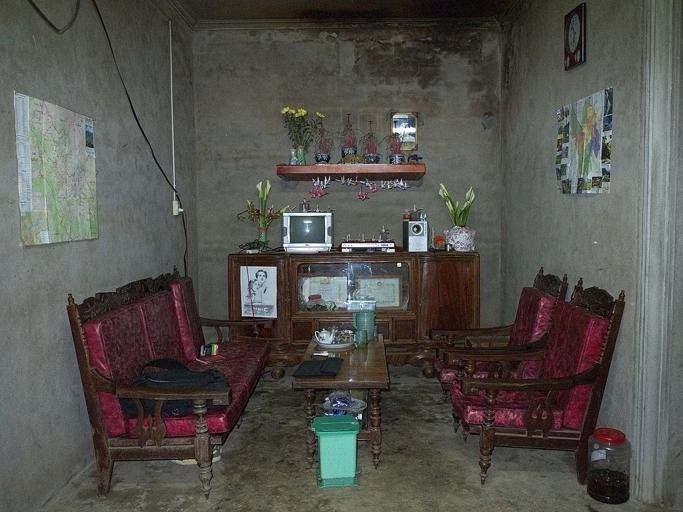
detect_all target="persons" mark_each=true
[244,279,260,302]
[254,268,268,292]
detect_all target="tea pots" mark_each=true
[311,328,335,345]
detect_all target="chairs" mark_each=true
[421,265,568,434]
[444,276,626,490]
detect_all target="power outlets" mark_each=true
[173,201,181,216]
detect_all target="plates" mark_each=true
[316,343,354,350]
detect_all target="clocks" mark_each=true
[563,2,585,71]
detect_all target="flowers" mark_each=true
[278,101,326,144]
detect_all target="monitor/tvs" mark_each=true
[283,213,332,251]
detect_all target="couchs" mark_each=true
[63,272,270,498]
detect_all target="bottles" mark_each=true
[354,310,376,342]
[584,428,632,506]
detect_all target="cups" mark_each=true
[335,329,367,347]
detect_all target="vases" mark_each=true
[446,225,479,253]
[296,145,306,165]
[257,219,268,245]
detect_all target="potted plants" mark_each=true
[339,111,356,156]
[437,184,477,227]
[387,132,407,164]
[362,118,382,163]
[313,118,332,164]
[234,177,291,217]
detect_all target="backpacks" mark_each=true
[122,356,232,418]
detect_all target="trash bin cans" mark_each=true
[308,415,362,490]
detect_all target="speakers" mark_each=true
[403,220,427,252]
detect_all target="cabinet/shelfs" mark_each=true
[224,248,480,368]
